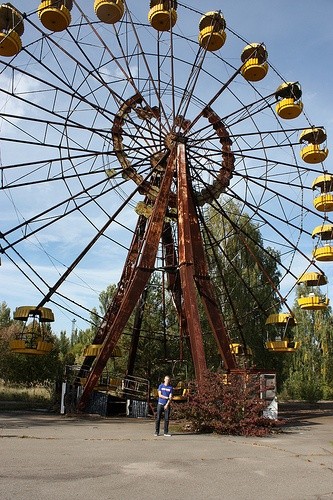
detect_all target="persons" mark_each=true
[153,375,174,437]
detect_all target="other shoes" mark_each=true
[154,431,159,436]
[164,434,172,436]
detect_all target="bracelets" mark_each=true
[168,397,169,399]
